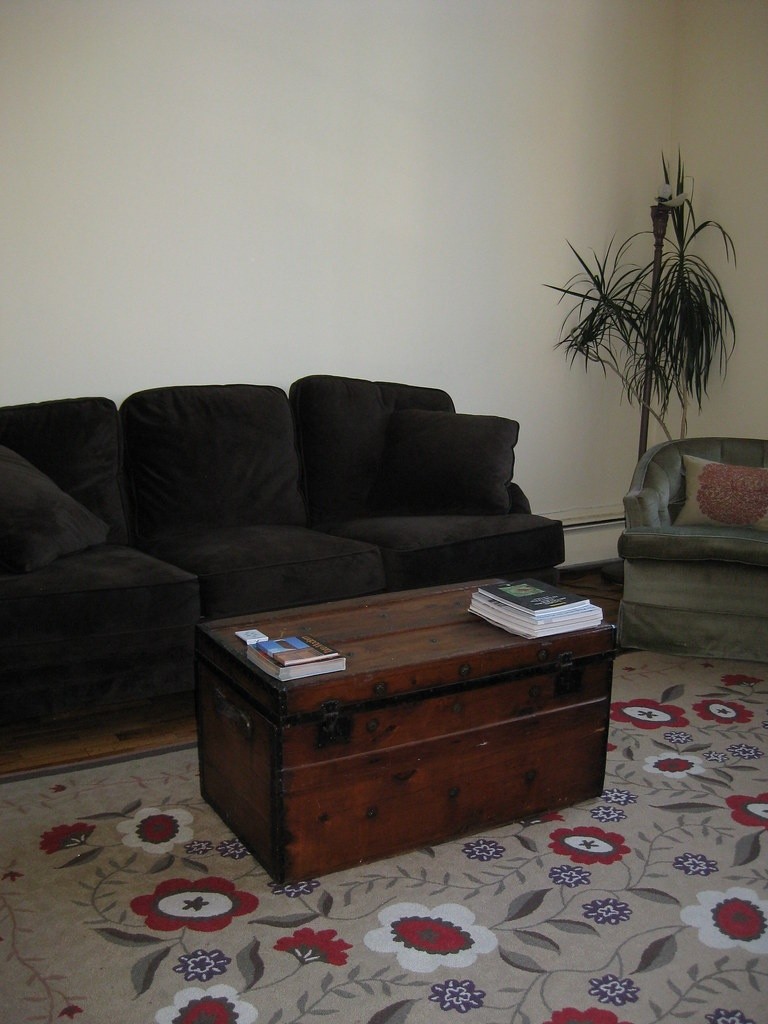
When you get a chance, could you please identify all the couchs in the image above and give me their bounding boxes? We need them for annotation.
[616,436,768,664]
[0,374,565,724]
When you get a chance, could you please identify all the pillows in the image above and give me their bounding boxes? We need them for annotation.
[364,410,520,516]
[0,444,111,574]
[669,455,768,533]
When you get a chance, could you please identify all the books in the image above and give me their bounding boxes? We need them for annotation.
[468,578,604,639]
[247,634,347,681]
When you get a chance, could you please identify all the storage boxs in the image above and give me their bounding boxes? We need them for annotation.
[196,577,618,883]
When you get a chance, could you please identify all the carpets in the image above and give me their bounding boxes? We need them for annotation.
[0,654,768,1024]
